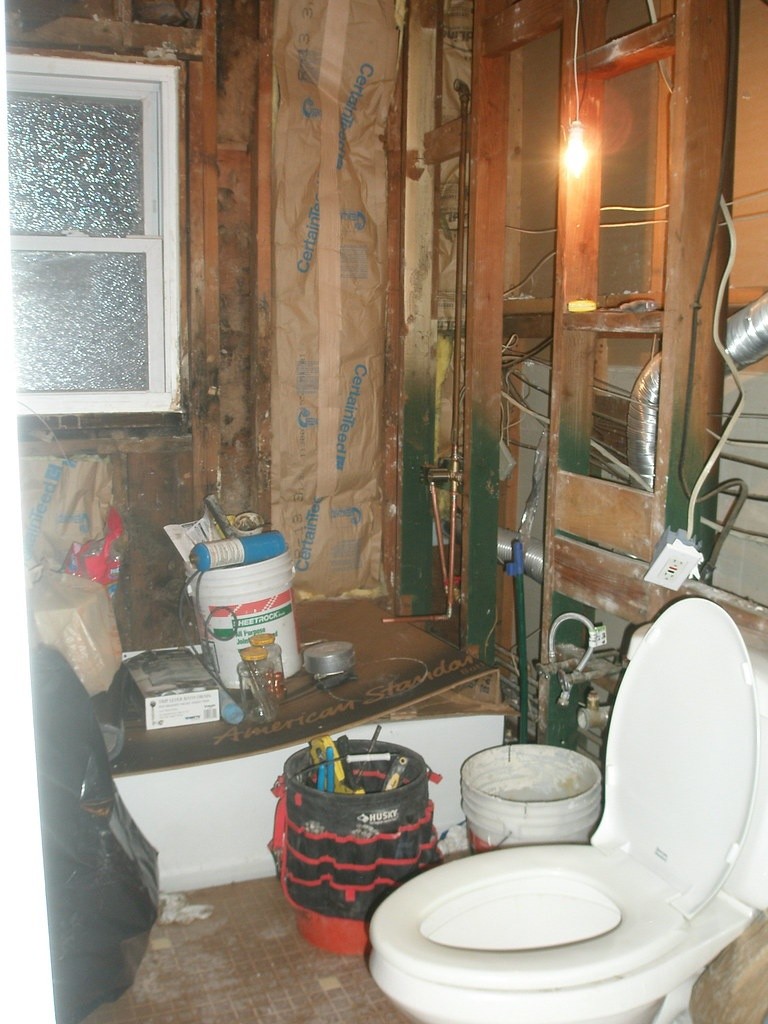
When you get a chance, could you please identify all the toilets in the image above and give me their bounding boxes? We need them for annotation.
[366,598,767,1021]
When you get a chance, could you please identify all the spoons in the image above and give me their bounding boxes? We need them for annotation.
[239,522,272,531]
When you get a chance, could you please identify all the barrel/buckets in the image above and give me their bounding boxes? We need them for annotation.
[282,726,444,956]
[460,744,602,855]
[185,550,302,690]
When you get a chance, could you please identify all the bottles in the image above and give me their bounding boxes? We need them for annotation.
[217,682,244,725]
[188,529,287,570]
[237,634,286,725]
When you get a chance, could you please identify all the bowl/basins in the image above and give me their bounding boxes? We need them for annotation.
[233,512,264,536]
